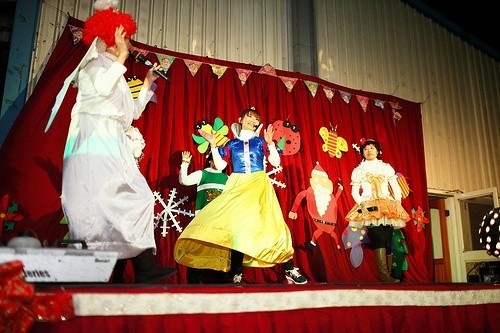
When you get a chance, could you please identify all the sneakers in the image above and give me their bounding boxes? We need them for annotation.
[284,265,307,284]
[231,269,242,286]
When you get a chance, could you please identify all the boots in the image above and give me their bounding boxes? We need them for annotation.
[375,248,400,283]
[386,255,392,275]
[112,259,127,283]
[131,249,176,283]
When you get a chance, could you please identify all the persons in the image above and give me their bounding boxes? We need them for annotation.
[350,139,411,283]
[173,109,307,287]
[45,8,178,284]
[178,149,230,284]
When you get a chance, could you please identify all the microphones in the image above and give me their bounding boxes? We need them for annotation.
[132,51,169,81]
